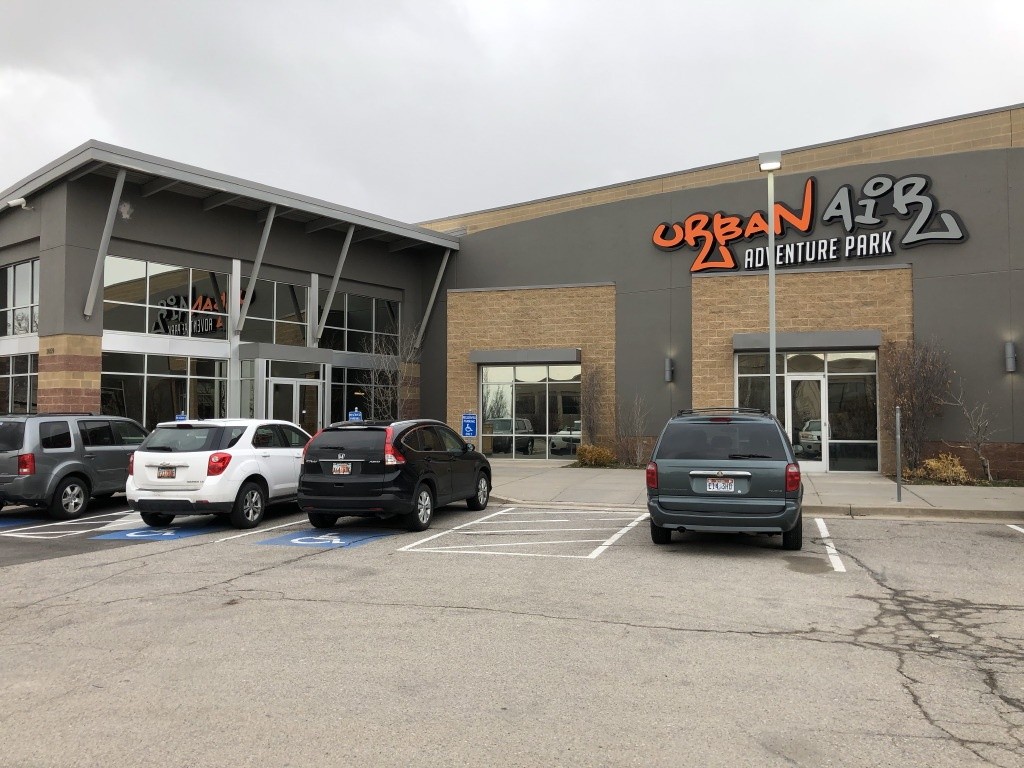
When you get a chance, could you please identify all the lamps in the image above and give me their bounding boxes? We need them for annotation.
[664,358,674,382]
[7,198,35,211]
[1005,342,1016,372]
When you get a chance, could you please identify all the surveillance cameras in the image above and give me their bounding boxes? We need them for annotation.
[8,198,26,208]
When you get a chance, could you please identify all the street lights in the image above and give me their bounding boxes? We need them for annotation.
[756,149,783,423]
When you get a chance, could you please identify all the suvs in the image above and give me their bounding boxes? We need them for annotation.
[295,419,492,532]
[645,407,804,552]
[485,418,536,455]
[126,419,312,530]
[0,412,152,519]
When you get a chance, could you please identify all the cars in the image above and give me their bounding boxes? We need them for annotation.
[798,419,833,453]
[550,421,583,456]
